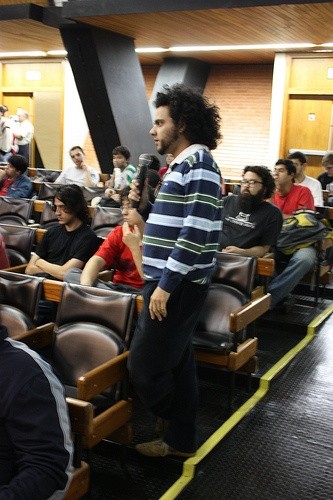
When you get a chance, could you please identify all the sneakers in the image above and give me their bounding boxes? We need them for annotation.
[135,438,196,457]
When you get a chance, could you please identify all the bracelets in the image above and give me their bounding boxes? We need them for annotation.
[33,258,41,266]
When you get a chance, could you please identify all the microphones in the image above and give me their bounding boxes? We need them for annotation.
[130,153,152,208]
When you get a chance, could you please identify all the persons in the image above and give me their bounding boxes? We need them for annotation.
[124,87,223,459]
[9,109,35,166]
[287,151,326,210]
[0,105,15,159]
[24,184,100,322]
[316,152,333,209]
[0,152,32,200]
[1,320,76,500]
[98,146,136,191]
[62,184,152,297]
[265,159,317,306]
[107,152,174,209]
[52,147,100,188]
[217,164,285,256]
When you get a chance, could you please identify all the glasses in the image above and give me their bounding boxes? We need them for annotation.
[272,168,288,174]
[239,179,263,187]
[120,203,136,211]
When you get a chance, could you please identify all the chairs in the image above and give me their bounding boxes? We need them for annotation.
[4,281,137,449]
[61,396,93,500]
[0,164,333,373]
